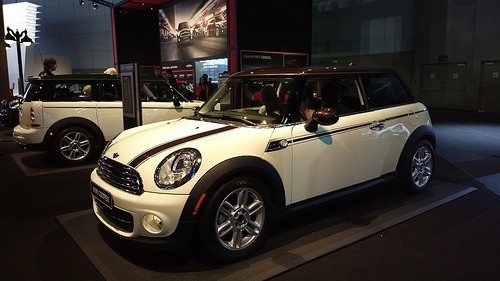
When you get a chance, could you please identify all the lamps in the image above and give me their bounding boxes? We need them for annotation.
[79,0,85,5]
[92,2,98,11]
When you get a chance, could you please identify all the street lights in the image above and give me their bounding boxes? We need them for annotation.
[2,27,34,97]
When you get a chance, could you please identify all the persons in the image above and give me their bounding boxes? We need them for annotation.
[152,68,164,80]
[259,85,317,122]
[38,57,57,76]
[166,70,178,90]
[196,74,211,101]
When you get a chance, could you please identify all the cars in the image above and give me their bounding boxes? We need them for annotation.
[0,93,24,128]
[89,66,438,263]
[175,15,227,48]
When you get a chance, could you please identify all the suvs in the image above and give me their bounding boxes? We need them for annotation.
[11,73,222,167]
